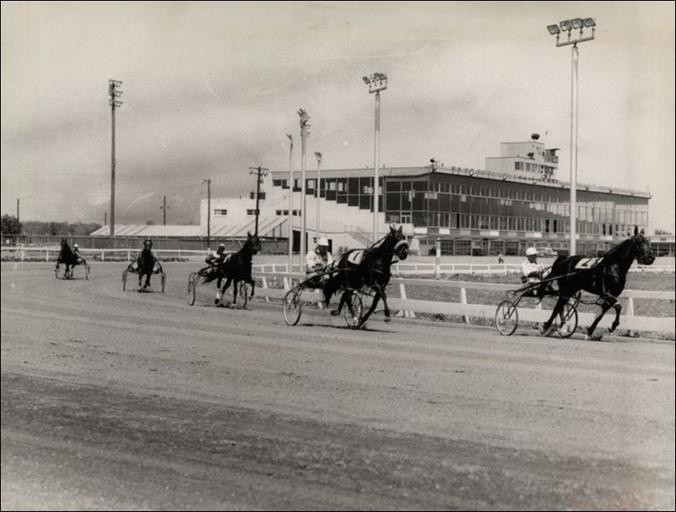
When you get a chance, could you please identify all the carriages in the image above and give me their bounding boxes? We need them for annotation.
[181,230,266,312]
[491,225,657,340]
[279,224,411,328]
[50,237,96,281]
[115,240,172,293]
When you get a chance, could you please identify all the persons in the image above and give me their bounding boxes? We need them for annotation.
[205,243,226,274]
[305,236,334,279]
[498,251,505,263]
[518,246,559,293]
[73,243,82,263]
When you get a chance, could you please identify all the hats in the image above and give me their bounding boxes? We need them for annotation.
[317,237,329,246]
[73,244,78,247]
[526,247,538,256]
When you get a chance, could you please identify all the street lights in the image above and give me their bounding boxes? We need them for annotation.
[284,132,294,275]
[544,16,604,260]
[296,106,311,280]
[105,77,122,237]
[314,149,325,246]
[362,71,389,248]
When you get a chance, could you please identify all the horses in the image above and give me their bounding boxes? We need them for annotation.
[137,238,154,291]
[323,223,410,328]
[202,230,259,307]
[54,237,78,279]
[535,225,655,340]
